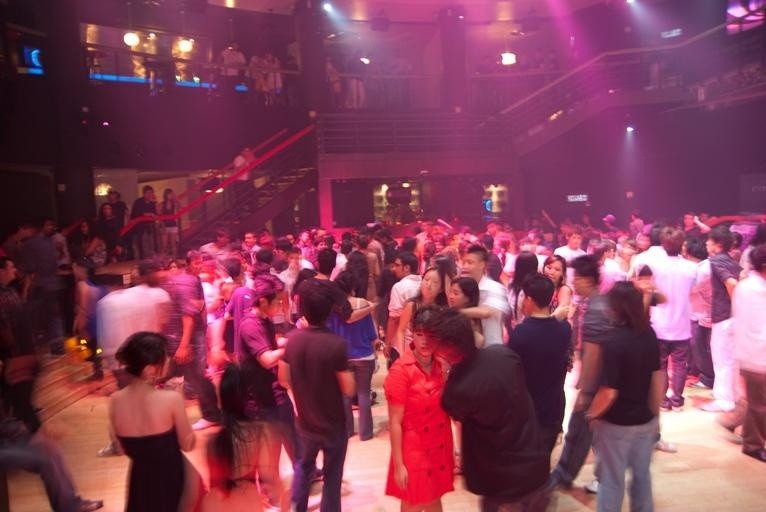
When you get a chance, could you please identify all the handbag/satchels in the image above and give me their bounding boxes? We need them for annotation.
[222,364,276,423]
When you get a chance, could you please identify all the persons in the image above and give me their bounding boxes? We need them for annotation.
[425,307,554,509]
[505,208,764,462]
[138,224,385,442]
[383,305,462,509]
[233,274,323,483]
[548,255,619,493]
[278,277,356,509]
[193,361,292,509]
[2,188,180,509]
[135,29,378,110]
[585,281,664,508]
[375,218,515,476]
[506,272,572,473]
[234,146,257,218]
[109,331,207,509]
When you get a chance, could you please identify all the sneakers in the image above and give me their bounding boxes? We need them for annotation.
[97,446,119,457]
[189,419,219,430]
[701,402,726,411]
[657,442,676,452]
[585,479,598,493]
[80,498,103,511]
[660,403,684,412]
